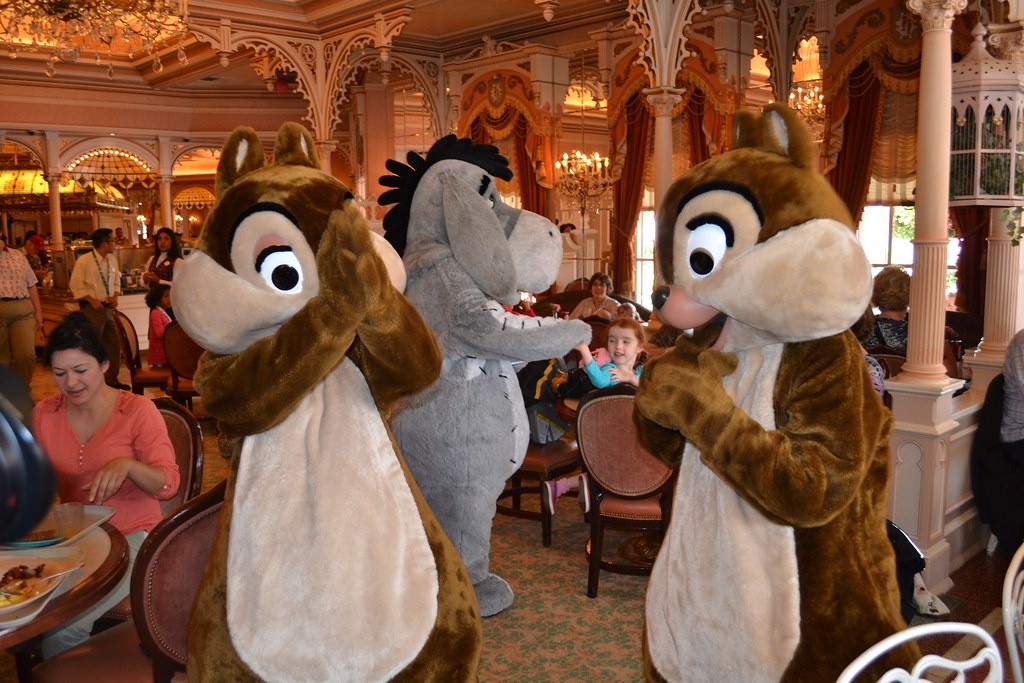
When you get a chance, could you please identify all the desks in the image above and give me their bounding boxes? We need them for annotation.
[0,523,130,678]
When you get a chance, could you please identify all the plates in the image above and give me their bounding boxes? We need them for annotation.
[0,505,116,628]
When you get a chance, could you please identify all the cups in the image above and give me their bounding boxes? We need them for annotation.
[53,502,84,539]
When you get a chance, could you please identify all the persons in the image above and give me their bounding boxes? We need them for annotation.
[137,227,189,389]
[113,227,130,246]
[964,330,1024,576]
[0,232,42,387]
[69,228,131,390]
[497,268,684,444]
[24,231,48,286]
[145,283,173,368]
[849,266,951,624]
[30,307,185,663]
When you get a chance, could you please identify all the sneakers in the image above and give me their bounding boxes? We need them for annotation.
[578,472,591,513]
[542,480,558,516]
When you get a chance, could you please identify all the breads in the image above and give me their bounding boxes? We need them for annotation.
[18,530,57,542]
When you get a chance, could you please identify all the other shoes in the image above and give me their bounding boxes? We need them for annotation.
[113,383,130,390]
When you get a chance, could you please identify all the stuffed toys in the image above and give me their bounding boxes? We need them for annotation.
[170,123,484,683]
[373,132,593,619]
[627,104,924,683]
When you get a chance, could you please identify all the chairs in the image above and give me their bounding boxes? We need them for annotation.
[100,398,206,622]
[109,309,171,394]
[161,320,219,431]
[574,382,685,597]
[495,440,592,546]
[30,478,228,683]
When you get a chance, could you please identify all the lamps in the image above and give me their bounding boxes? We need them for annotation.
[0,0,190,79]
[556,151,608,220]
[789,86,826,125]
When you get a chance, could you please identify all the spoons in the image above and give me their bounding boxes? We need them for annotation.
[0,563,86,595]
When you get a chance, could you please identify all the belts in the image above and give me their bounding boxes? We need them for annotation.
[1,297,19,301]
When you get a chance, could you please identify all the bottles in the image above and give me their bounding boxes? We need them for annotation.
[560,312,571,321]
